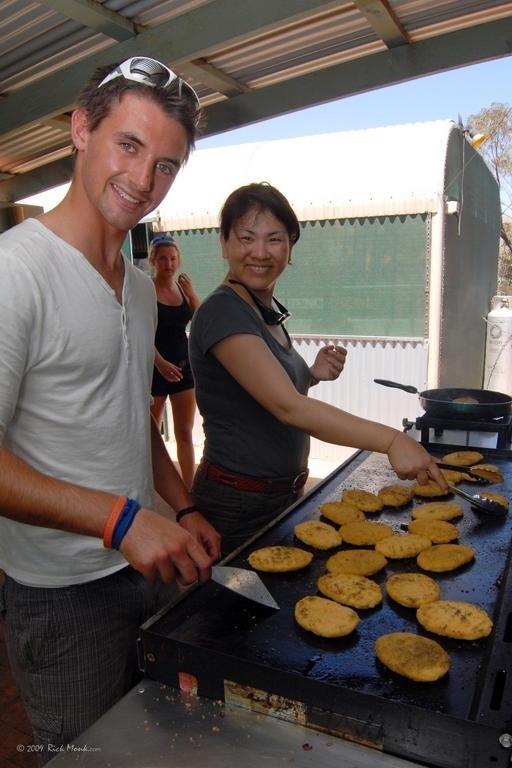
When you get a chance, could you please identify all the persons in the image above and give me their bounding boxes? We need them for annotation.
[189,182,449,557]
[0,54,222,768]
[149,235,202,489]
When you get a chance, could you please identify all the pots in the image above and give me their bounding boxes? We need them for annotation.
[372,378,512,419]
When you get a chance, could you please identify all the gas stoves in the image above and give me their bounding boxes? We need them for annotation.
[415,412,512,449]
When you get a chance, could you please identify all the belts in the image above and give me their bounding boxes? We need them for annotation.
[197,456,309,495]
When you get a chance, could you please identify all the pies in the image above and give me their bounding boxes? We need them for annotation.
[453,397,478,405]
[247,450,509,682]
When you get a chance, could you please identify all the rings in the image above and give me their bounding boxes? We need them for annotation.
[170,372,174,374]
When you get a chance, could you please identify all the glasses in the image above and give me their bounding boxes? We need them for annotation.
[96,56,203,127]
[228,279,291,327]
[151,236,176,245]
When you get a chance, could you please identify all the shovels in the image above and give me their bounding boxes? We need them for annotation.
[195,566,280,609]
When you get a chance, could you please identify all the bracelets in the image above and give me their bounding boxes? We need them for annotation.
[386,431,400,453]
[176,506,198,521]
[112,499,142,551]
[103,495,127,548]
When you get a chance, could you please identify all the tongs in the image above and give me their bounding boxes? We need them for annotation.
[426,462,508,519]
